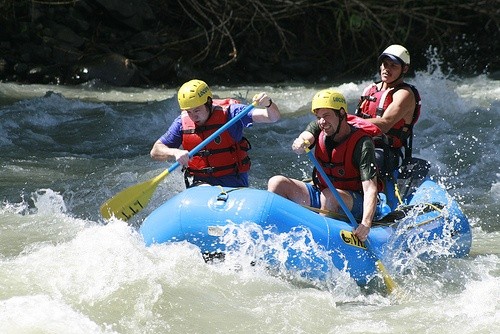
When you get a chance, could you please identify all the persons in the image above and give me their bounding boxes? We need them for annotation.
[150,78,280,187]
[355,44,421,201]
[267,90,377,241]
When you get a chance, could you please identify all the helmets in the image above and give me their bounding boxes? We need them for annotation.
[310,89,348,115]
[382,45,412,62]
[178,79,213,109]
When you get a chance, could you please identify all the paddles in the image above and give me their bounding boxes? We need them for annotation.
[302,146,402,301]
[98,100,262,224]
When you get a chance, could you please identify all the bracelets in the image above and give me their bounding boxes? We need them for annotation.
[266,99,272,107]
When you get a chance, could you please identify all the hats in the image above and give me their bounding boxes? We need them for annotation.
[377,54,401,64]
